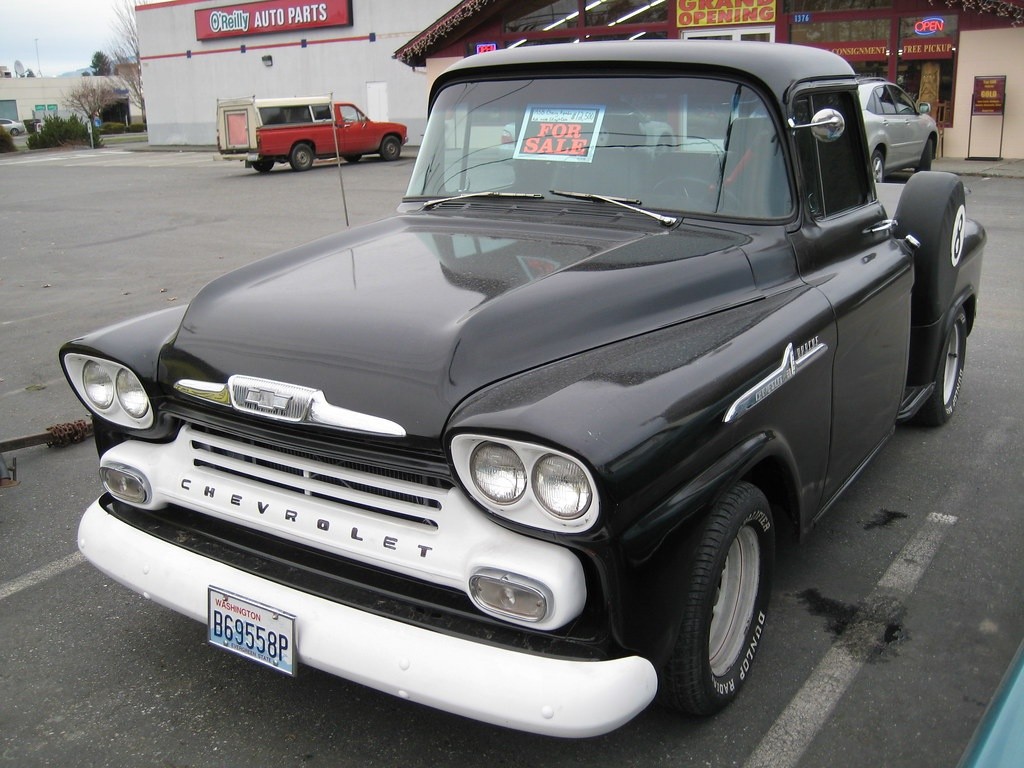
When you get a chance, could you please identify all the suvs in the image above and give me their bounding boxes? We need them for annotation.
[60,37,987,741]
[852,77,941,183]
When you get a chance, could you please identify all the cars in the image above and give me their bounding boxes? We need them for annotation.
[0,118,25,136]
[497,96,675,180]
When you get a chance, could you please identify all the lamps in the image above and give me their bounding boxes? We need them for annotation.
[262,56,272,66]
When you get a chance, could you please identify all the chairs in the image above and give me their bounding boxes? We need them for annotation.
[707,117,791,218]
[552,115,650,197]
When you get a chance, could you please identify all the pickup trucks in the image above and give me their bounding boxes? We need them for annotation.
[215,91,408,171]
